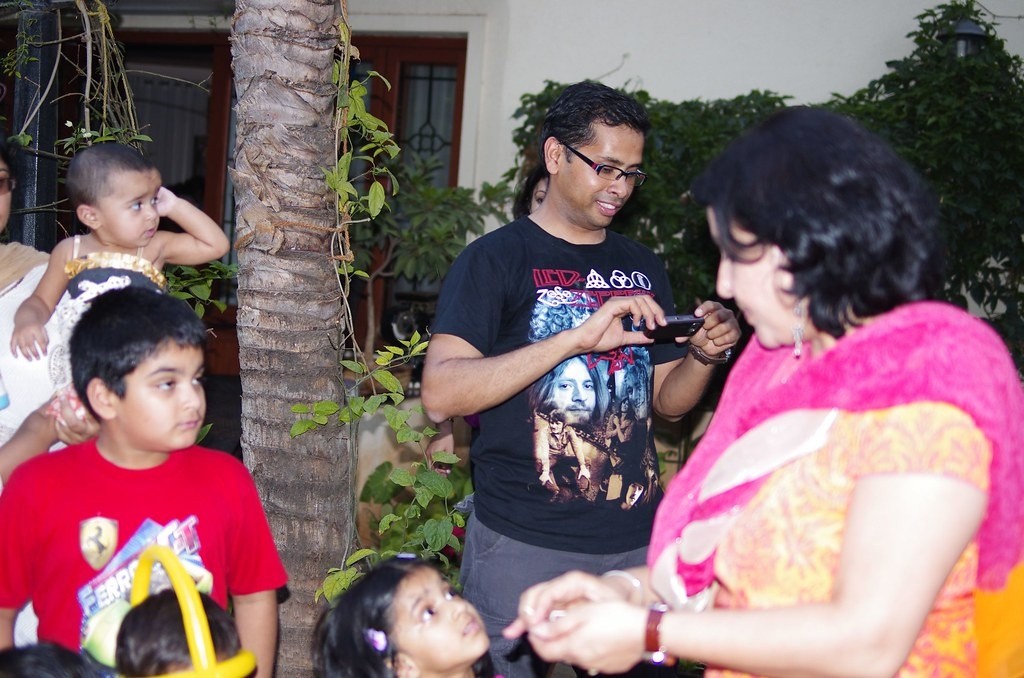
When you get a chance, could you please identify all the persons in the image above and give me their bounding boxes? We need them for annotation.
[0,80,1024,678]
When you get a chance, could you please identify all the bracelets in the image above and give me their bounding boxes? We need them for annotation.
[602,570,647,609]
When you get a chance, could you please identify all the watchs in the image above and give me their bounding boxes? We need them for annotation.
[642,604,676,668]
[688,342,732,366]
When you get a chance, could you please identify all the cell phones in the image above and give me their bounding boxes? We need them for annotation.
[643,314,705,339]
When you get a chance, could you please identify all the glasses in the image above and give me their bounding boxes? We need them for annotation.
[557,138,648,187]
